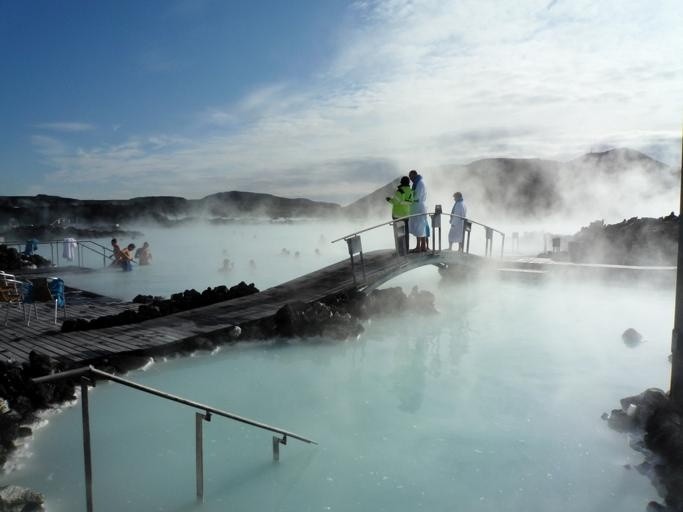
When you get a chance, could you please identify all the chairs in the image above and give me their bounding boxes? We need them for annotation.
[17,276,65,325]
[6,279,34,327]
[0,270,23,325]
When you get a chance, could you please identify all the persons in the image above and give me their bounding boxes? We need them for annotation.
[108,233,326,277]
[384,175,413,257]
[408,171,429,252]
[445,191,468,251]
